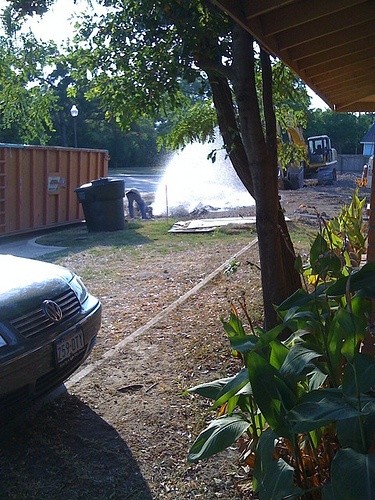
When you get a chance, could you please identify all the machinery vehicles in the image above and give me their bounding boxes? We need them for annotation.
[289,135,339,189]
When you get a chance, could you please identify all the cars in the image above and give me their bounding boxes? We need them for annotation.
[0,253,103,412]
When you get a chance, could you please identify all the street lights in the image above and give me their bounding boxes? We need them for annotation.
[70,104,78,148]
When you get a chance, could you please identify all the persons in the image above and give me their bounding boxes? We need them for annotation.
[315,145,323,155]
[126,187,153,219]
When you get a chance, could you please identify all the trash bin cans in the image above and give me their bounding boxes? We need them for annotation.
[76,177,126,233]
